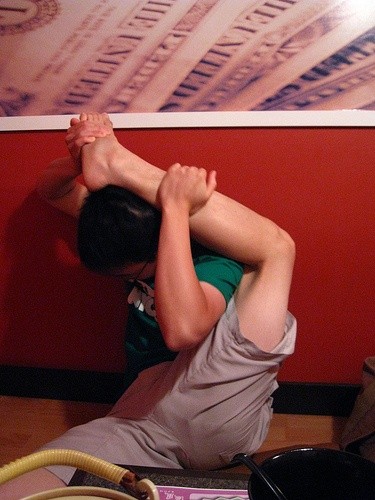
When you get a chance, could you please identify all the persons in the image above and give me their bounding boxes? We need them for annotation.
[0,111,298,499]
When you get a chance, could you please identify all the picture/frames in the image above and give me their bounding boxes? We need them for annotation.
[1,0,375,133]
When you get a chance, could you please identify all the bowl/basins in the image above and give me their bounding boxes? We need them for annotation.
[249,446,374,500]
